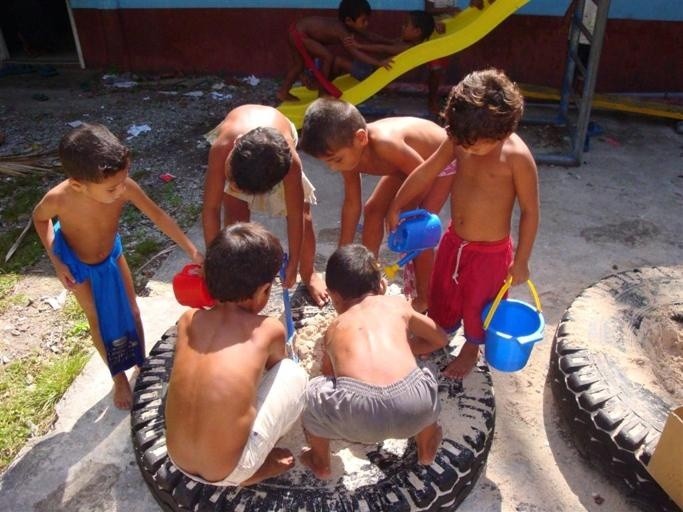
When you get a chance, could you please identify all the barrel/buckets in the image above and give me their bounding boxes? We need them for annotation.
[481,276,545,372]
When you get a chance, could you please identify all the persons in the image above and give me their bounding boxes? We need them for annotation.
[276,0,395,100]
[564,0,607,98]
[329,10,436,84]
[468,0,506,9]
[386,69,539,378]
[298,244,448,478]
[299,57,323,85]
[298,96,458,312]
[32,123,205,409]
[165,223,310,487]
[423,0,461,117]
[202,103,332,306]
